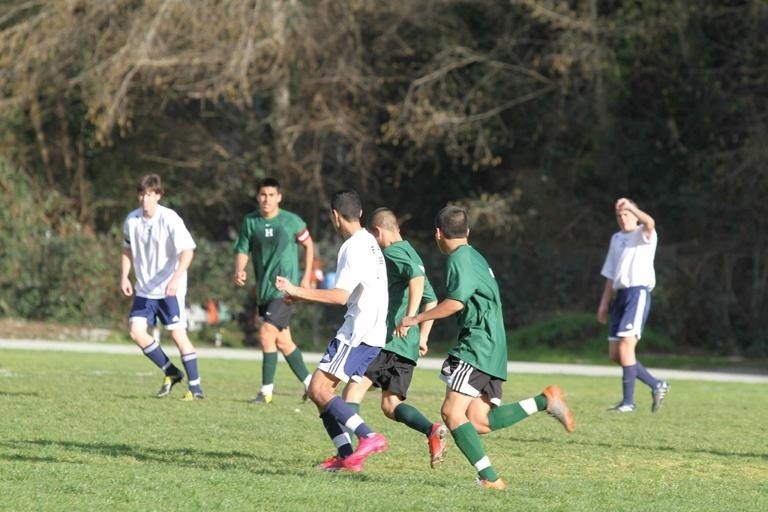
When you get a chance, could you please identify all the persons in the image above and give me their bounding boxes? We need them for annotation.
[342,206,448,470]
[597,197,671,413]
[274,189,389,471]
[232,178,314,404]
[401,205,574,488]
[119,172,204,400]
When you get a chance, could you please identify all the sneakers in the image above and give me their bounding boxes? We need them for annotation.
[427,421,449,470]
[608,402,636,414]
[651,379,670,413]
[157,369,185,400]
[342,432,388,468]
[248,391,274,405]
[470,477,506,492]
[542,383,574,433]
[315,456,362,472]
[178,390,204,402]
[300,390,312,405]
